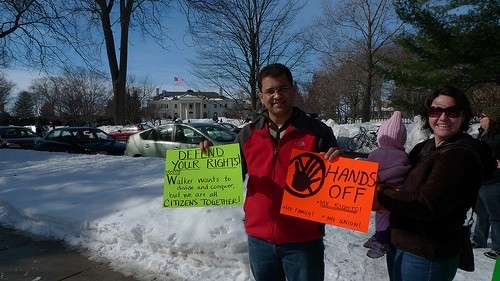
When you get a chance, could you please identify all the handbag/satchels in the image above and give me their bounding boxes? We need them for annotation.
[457,219,474,272]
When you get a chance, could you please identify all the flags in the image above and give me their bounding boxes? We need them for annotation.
[174,76,184,86]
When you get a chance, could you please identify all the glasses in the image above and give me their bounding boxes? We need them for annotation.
[262,85,293,96]
[480,114,487,118]
[425,106,464,119]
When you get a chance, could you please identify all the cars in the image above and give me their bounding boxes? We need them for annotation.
[0,120,241,157]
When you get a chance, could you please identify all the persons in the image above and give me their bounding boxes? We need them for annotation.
[353,109,409,260]
[239,115,252,124]
[133,112,191,143]
[213,111,219,123]
[464,107,500,260]
[385,87,497,281]
[33,120,56,138]
[198,63,343,281]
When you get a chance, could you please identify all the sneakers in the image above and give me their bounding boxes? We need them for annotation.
[471,239,484,248]
[367,242,388,259]
[483,249,500,259]
[363,233,380,248]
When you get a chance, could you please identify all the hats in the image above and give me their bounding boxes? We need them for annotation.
[376,111,408,151]
[482,106,500,120]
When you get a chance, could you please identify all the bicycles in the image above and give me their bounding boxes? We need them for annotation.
[347,125,379,150]
